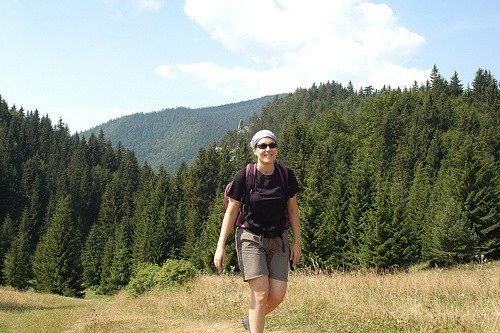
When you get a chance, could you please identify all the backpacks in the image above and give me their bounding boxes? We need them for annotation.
[224,162,289,228]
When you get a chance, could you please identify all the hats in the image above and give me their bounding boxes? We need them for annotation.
[249,129,278,148]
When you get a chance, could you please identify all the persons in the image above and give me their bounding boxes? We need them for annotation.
[212,129,302,333]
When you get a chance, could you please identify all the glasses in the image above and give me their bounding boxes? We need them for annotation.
[256,143,277,149]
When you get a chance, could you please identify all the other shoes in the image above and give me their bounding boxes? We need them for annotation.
[243,316,249,331]
